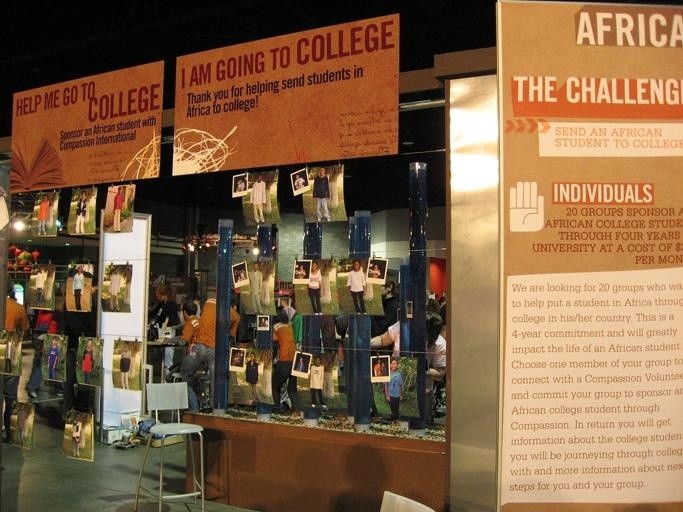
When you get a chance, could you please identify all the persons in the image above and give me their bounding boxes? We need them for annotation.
[145,282,240,412]
[5,186,132,458]
[229,275,448,421]
[234,262,263,314]
[293,168,331,221]
[295,261,324,315]
[234,173,266,224]
[346,261,381,314]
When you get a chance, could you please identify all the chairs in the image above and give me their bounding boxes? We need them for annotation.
[379,490,436,512]
[133,382,205,512]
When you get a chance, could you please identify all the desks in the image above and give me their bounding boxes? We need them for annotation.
[147,340,189,383]
